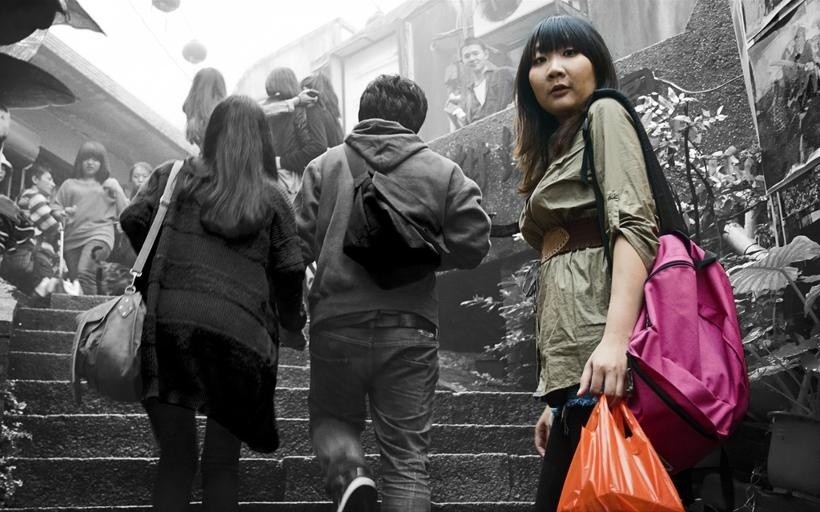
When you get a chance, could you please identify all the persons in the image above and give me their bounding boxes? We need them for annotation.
[0,108,154,331]
[443,44,531,129]
[182,68,319,158]
[259,67,331,185]
[292,74,492,511]
[508,13,699,510]
[120,95,309,512]
[265,72,347,173]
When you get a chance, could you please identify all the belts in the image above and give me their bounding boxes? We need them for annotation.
[347,311,437,330]
[538,221,602,264]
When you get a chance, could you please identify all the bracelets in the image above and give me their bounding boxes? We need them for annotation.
[297,96,304,107]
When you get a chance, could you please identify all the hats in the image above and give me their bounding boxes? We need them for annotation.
[0,153,13,172]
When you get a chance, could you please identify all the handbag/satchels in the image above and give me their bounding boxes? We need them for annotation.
[1,250,35,276]
[72,293,144,403]
[343,170,448,290]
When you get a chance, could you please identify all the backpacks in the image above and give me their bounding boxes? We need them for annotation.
[582,88,750,477]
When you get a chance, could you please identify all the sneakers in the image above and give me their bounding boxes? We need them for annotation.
[335,468,378,512]
